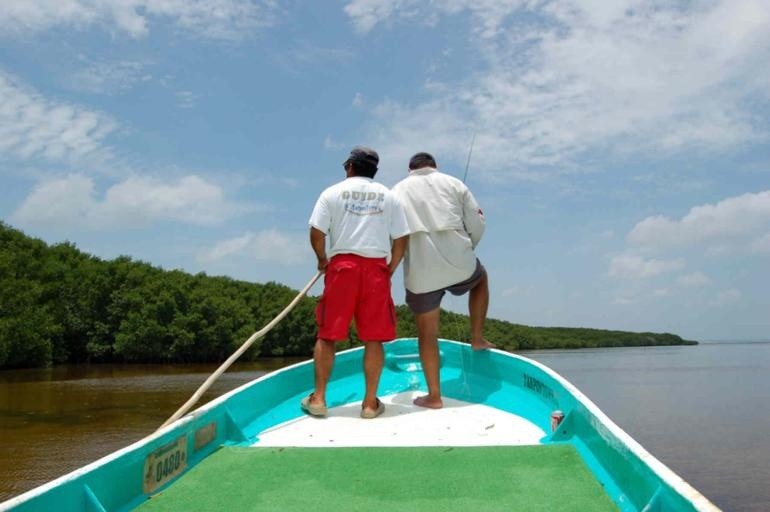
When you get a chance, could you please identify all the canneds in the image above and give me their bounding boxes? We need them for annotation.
[551,410,564,432]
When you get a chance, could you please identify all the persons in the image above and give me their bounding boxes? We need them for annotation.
[301,146,411,419]
[387,153,497,408]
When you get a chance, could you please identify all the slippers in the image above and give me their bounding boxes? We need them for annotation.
[300,393,327,417]
[360,397,385,419]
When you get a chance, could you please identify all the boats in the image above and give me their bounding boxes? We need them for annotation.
[0,334,723,512]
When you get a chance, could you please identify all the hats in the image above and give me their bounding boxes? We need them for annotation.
[342,146,379,171]
